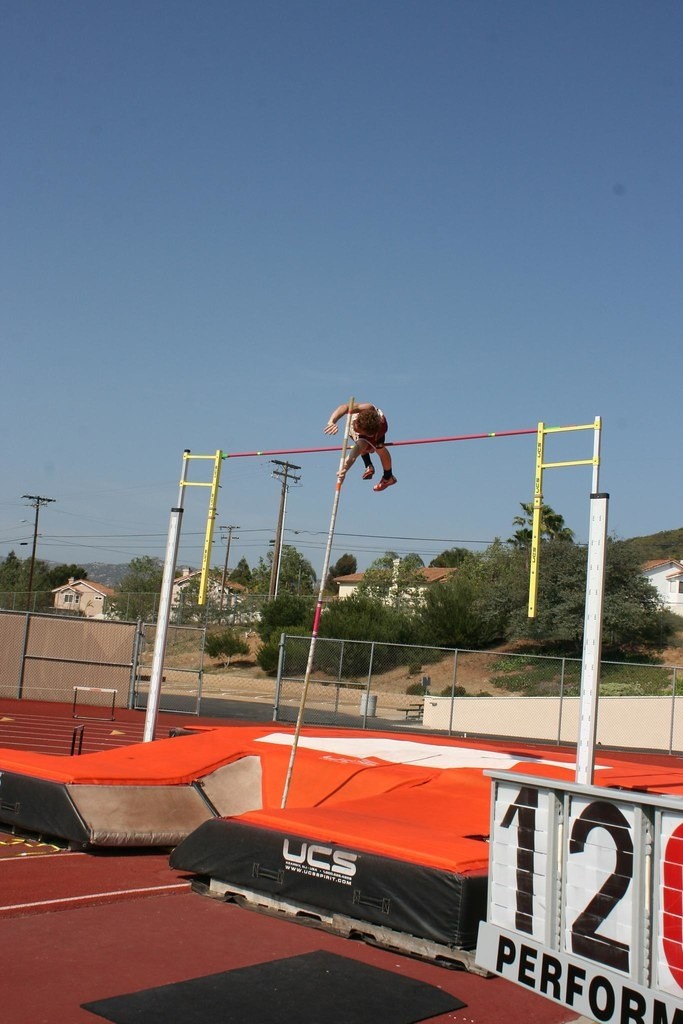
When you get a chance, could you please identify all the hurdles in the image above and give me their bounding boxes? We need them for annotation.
[69,725,86,756]
[71,685,118,720]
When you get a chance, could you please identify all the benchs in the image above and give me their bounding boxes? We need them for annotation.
[396,708,424,719]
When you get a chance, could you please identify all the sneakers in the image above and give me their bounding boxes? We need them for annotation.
[373,475,397,491]
[362,466,375,480]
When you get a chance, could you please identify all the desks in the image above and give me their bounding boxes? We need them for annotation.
[409,704,424,716]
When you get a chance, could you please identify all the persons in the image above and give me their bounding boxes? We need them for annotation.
[325,402,398,492]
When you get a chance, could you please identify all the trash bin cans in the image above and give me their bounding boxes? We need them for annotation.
[359,693,378,717]
